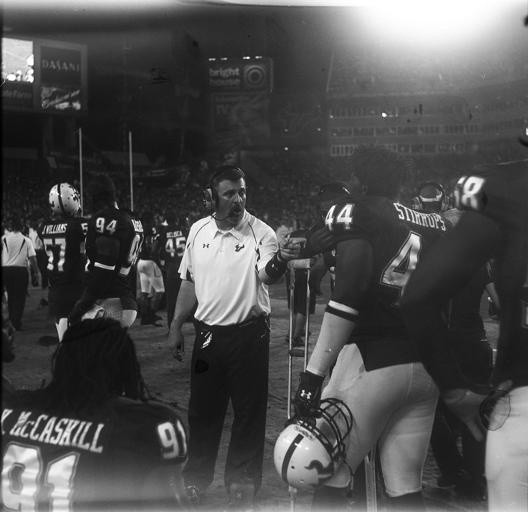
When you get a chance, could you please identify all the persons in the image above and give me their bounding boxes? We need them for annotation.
[150,210,190,337]
[292,143,445,508]
[67,174,144,331]
[445,123,528,508]
[0,301,17,403]
[135,212,166,326]
[0,317,188,507]
[422,259,496,501]
[32,182,89,343]
[1,216,41,333]
[284,211,325,348]
[167,164,303,507]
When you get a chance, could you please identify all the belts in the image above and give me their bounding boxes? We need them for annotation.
[193,321,258,335]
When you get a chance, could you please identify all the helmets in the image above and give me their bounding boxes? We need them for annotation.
[273,396,354,492]
[49,181,81,218]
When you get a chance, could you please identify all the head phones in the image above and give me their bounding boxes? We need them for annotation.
[201,166,237,223]
